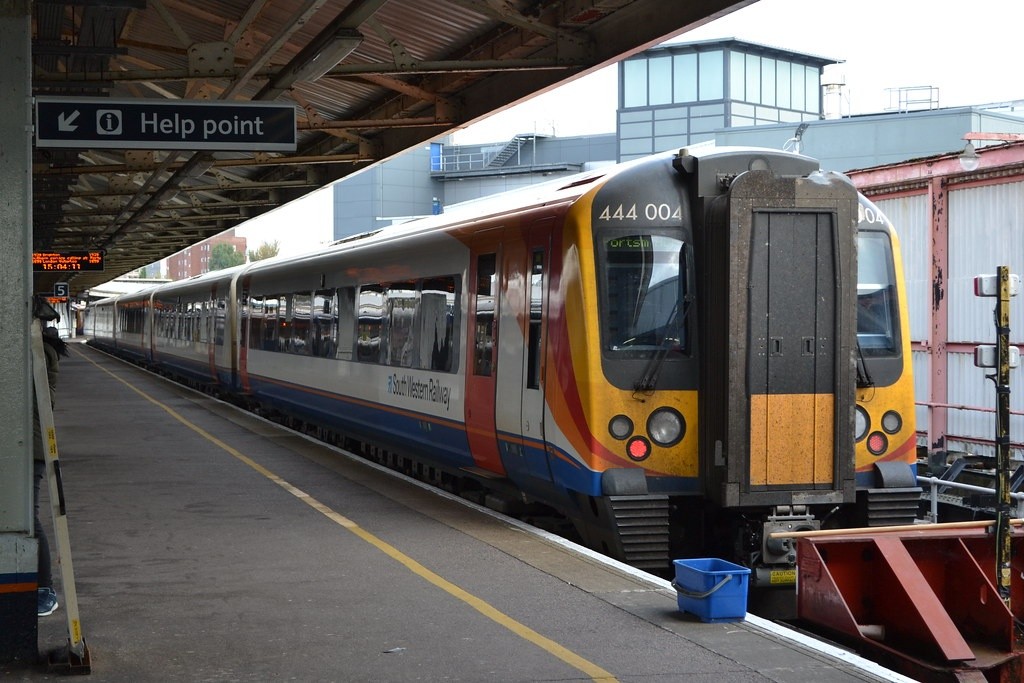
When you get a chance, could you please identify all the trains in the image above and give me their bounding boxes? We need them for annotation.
[83,148,924,622]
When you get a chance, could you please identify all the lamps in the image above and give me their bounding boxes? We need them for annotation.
[292,27,364,83]
[960,133,1024,171]
[106,209,156,249]
[156,183,181,200]
[186,155,216,179]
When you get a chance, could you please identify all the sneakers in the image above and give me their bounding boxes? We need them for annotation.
[37,588,58,616]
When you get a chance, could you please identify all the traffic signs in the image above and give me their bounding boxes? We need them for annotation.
[34,94,297,150]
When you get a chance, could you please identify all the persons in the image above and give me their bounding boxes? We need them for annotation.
[33,295,69,617]
